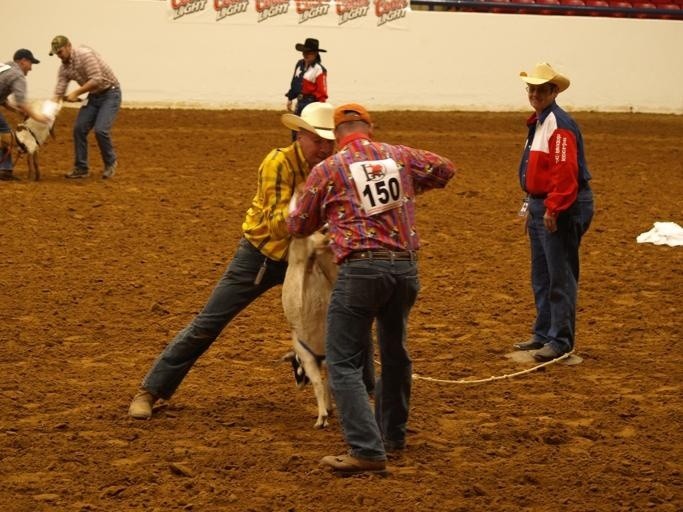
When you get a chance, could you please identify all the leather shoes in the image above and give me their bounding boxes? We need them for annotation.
[0,170,20,180]
[129,390,154,417]
[513,339,543,349]
[323,454,386,470]
[534,344,558,360]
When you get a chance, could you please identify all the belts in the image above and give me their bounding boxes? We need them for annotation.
[348,253,416,259]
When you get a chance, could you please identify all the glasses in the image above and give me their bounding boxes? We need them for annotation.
[526,86,549,94]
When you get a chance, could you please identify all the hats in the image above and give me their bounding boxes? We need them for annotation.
[296,38,326,52]
[334,104,371,126]
[49,36,68,55]
[281,102,336,140]
[13,48,40,63]
[520,62,570,92]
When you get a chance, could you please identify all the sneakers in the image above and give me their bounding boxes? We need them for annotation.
[103,160,117,178]
[65,169,89,178]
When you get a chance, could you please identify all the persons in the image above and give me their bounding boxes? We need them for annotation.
[284,103,456,475]
[283,36,328,142]
[44,32,123,180]
[127,101,379,421]
[512,60,594,362]
[0,46,50,184]
